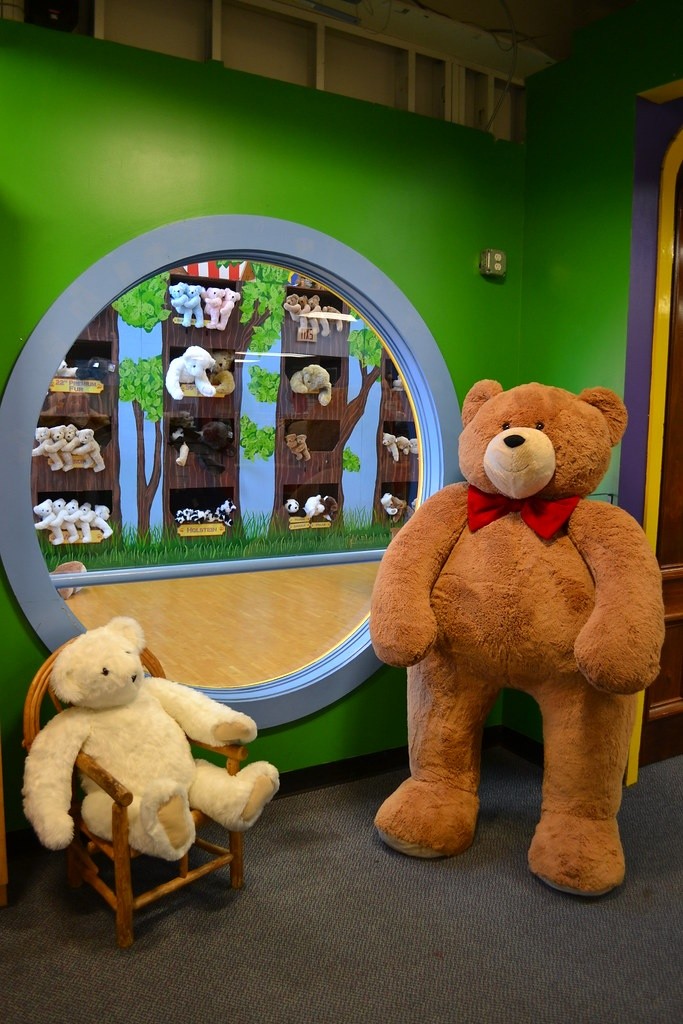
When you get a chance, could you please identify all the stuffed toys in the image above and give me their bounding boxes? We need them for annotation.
[290,365,332,406]
[168,282,192,327]
[31,412,407,545]
[308,295,330,337]
[283,294,301,322]
[184,283,203,328]
[56,357,111,380]
[382,433,399,461]
[208,350,235,394]
[298,295,319,334]
[322,306,343,331]
[395,436,410,456]
[201,287,224,329]
[372,378,666,897]
[21,618,280,861]
[409,439,419,454]
[49,561,87,599]
[167,346,216,399]
[218,288,241,330]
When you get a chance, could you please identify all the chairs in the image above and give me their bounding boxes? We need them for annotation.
[22,632,248,948]
